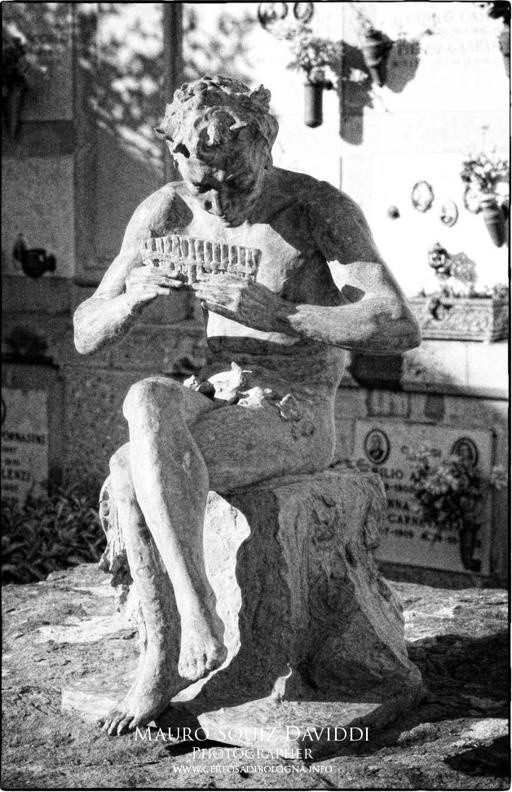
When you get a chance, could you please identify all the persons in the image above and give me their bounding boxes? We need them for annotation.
[72,76,423,735]
